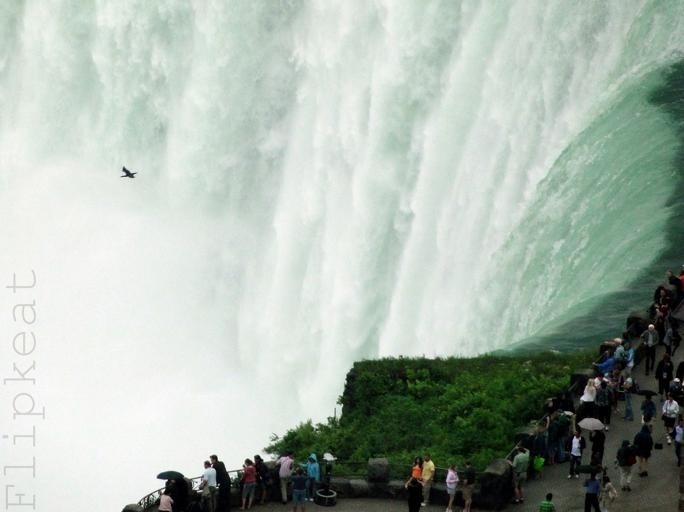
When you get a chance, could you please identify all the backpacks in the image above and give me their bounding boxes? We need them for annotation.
[643,401,653,422]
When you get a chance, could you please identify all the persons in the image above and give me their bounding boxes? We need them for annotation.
[505,266,683,511]
[154,448,476,511]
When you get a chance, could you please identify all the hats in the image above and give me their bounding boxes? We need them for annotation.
[673,378,680,382]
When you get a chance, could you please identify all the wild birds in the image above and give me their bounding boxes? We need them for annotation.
[121,166,137,179]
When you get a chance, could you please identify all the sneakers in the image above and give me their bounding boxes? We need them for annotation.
[567,473,580,479]
[511,496,525,504]
[420,501,431,507]
[638,471,649,478]
[622,487,632,492]
[445,508,471,512]
